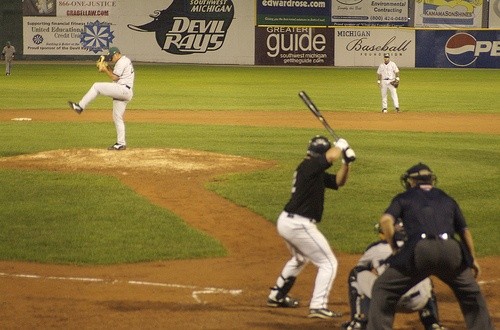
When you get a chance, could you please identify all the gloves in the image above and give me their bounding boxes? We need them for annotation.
[333,138,348,151]
[341,149,356,165]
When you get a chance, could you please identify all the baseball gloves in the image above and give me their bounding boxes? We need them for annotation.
[390,79,399,88]
[96,55,109,73]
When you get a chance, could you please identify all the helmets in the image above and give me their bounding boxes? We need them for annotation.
[306,136,331,158]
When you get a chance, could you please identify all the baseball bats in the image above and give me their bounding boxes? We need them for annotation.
[297,91,356,162]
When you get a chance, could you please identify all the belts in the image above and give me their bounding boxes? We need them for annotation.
[126,85,131,89]
[416,233,449,240]
[287,213,293,217]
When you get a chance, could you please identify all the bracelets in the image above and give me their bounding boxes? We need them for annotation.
[396,77,400,81]
[377,80,380,83]
[378,79,380,80]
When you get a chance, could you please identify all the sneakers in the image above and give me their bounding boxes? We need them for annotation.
[68,101,83,114]
[308,309,342,319]
[108,143,126,150]
[266,295,298,307]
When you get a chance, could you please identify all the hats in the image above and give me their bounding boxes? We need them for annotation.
[385,54,389,57]
[107,47,120,59]
[403,162,431,177]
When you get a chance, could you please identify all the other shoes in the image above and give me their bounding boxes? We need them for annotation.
[396,108,400,112]
[382,108,386,112]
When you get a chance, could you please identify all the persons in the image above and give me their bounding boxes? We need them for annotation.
[68,47,134,150]
[342,217,450,330]
[366,162,493,330]
[267,135,357,319]
[377,53,401,113]
[2,41,15,75]
